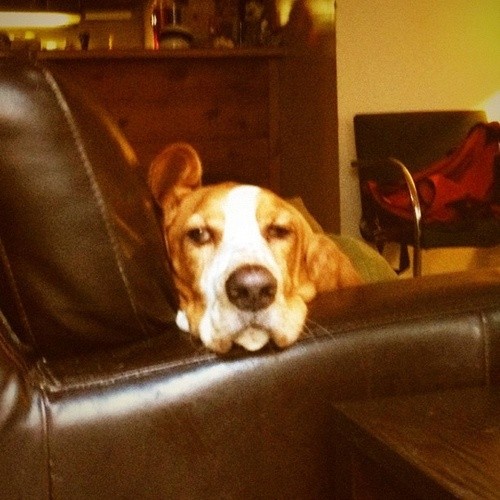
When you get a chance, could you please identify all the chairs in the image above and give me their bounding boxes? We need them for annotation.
[348,110,500,282]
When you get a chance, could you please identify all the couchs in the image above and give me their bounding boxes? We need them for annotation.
[0,55,500,500]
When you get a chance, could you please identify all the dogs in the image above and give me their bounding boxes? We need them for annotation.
[149,139,401,354]
